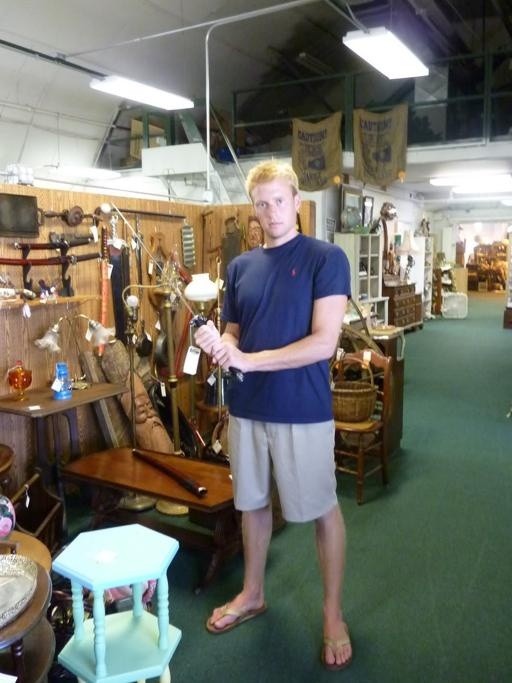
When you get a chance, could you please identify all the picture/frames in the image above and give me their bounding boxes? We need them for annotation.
[362,194,373,228]
[338,182,363,232]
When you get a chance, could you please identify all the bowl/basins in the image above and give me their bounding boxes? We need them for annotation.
[0,287,17,298]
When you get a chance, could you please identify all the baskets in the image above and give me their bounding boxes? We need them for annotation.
[330,357,378,423]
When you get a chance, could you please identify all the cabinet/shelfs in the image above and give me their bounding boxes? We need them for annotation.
[340,322,403,461]
[0,526,56,682]
[0,379,132,537]
[51,524,186,683]
[330,226,435,337]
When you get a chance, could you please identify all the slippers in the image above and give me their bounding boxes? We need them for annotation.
[206,599,266,634]
[321,621,353,671]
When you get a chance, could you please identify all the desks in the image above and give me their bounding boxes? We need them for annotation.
[62,443,245,596]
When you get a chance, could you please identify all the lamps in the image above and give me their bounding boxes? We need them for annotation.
[28,307,116,391]
[87,69,197,115]
[179,272,222,371]
[341,1,429,84]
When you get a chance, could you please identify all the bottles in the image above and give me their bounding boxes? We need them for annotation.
[53,361,73,400]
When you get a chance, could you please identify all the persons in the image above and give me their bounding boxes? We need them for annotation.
[195,158,353,671]
[119,370,174,453]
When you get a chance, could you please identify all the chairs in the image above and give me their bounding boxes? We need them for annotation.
[334,345,394,506]
